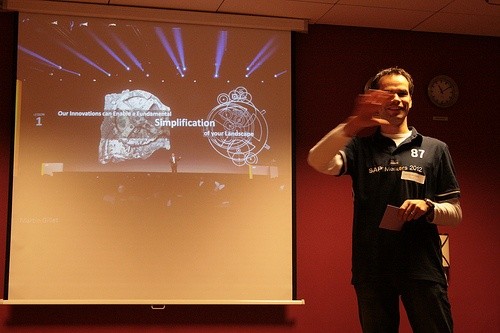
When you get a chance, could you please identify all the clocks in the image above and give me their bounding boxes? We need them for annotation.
[427,74,459,108]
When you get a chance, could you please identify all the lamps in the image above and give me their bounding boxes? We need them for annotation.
[438,234,451,289]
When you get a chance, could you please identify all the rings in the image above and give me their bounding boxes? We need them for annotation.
[411,212,415,216]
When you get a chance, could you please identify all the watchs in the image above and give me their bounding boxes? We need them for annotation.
[424,198,435,218]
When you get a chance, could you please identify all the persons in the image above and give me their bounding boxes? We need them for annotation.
[307,67,462,333]
